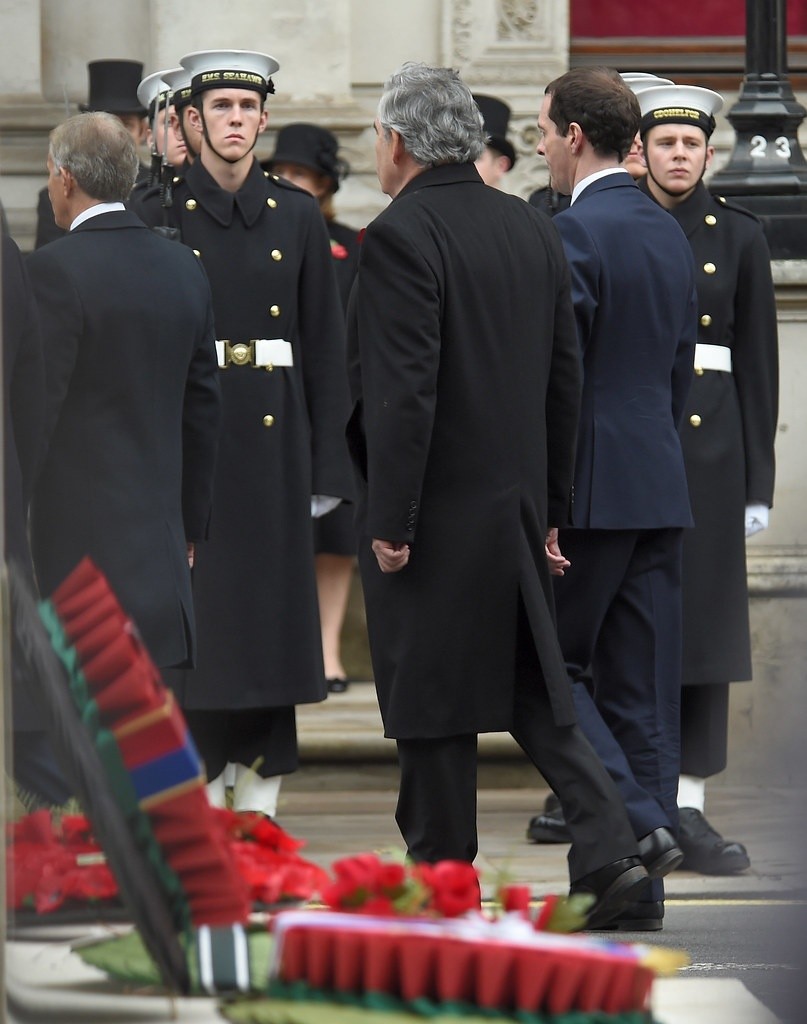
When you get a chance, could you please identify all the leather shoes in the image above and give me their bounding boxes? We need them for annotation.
[527,814,571,843]
[677,805,750,870]
[611,901,664,932]
[566,856,647,930]
[638,829,685,875]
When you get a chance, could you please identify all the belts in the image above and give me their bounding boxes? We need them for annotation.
[215,339,295,369]
[695,342,733,374]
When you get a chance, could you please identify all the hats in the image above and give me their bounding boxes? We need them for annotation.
[259,124,339,176]
[635,84,725,137]
[137,71,174,122]
[79,59,149,118]
[471,95,515,173]
[623,77,674,95]
[161,68,195,112]
[179,50,279,106]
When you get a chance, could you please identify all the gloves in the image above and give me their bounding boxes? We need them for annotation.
[312,496,343,519]
[745,502,769,539]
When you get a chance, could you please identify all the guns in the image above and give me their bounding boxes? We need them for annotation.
[153,90,180,241]
[146,85,163,188]
[546,176,561,218]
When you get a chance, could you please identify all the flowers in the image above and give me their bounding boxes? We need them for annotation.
[11,552,662,1024]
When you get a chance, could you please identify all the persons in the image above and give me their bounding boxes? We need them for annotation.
[2,49,782,928]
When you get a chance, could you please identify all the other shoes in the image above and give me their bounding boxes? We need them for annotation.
[329,679,348,693]
